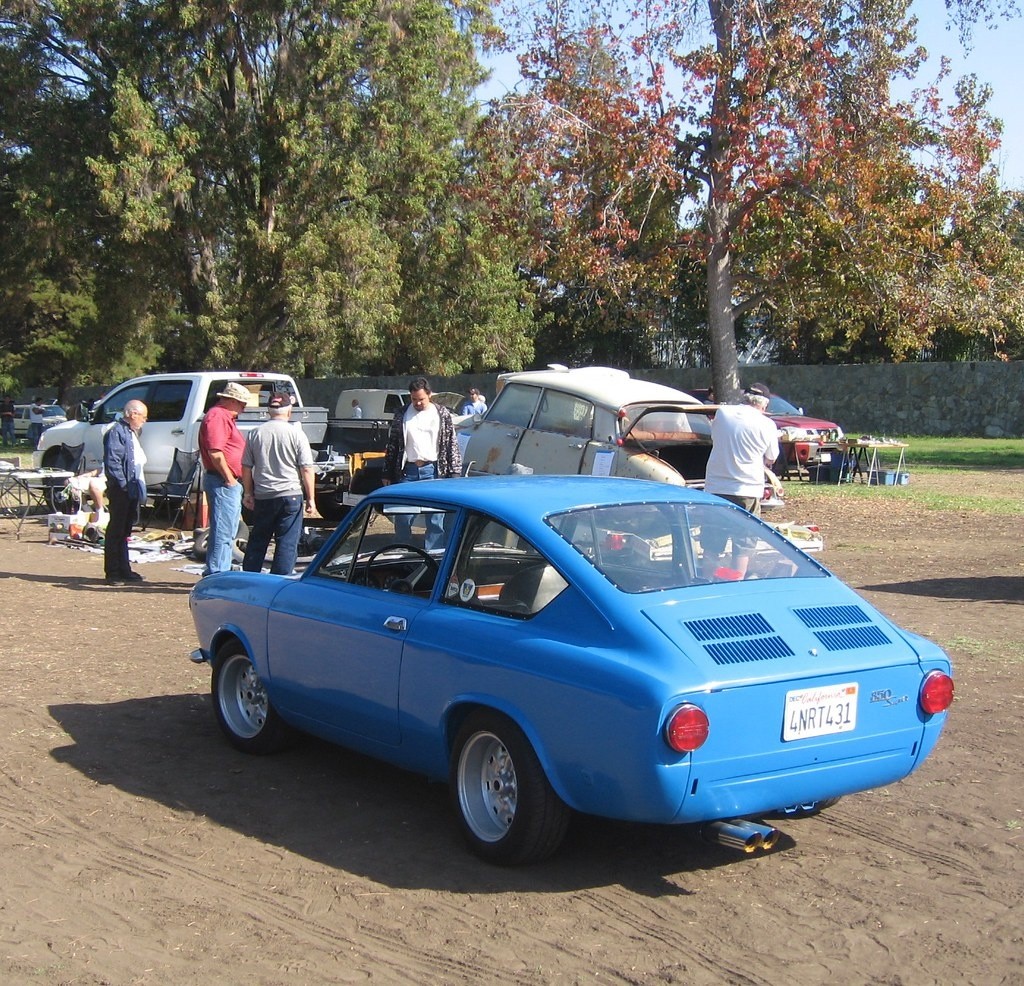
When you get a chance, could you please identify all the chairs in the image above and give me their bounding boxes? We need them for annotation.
[31,442,85,515]
[0,457,24,505]
[73,481,109,514]
[140,447,200,532]
[499,563,569,615]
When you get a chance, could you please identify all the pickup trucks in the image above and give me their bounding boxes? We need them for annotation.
[35,372,390,524]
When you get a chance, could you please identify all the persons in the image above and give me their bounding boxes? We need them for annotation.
[0,395,17,443]
[64,399,148,586]
[352,399,362,418]
[459,388,487,415]
[30,397,43,450]
[382,377,462,550]
[198,382,250,577]
[241,393,316,575]
[699,383,780,580]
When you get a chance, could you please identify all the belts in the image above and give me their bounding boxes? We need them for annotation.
[405,460,438,467]
[207,469,220,476]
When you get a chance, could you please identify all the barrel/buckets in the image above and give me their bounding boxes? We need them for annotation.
[829,452,855,483]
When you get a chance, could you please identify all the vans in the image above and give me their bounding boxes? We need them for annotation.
[336,389,411,419]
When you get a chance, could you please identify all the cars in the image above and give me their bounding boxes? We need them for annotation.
[189,476,954,870]
[689,387,846,476]
[460,374,782,522]
[13,405,67,437]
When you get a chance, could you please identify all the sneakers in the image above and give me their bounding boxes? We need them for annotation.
[105,571,144,586]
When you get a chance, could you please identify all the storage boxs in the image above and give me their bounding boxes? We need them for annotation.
[242,384,262,408]
[183,492,209,531]
[808,466,826,481]
[77,511,110,539]
[894,474,909,485]
[865,470,894,485]
[781,434,792,441]
[48,514,84,540]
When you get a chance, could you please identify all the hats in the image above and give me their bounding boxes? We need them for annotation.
[468,387,480,396]
[744,382,771,400]
[267,392,291,409]
[216,382,251,404]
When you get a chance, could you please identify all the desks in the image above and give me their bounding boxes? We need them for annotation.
[779,439,803,481]
[837,444,909,487]
[0,469,75,540]
